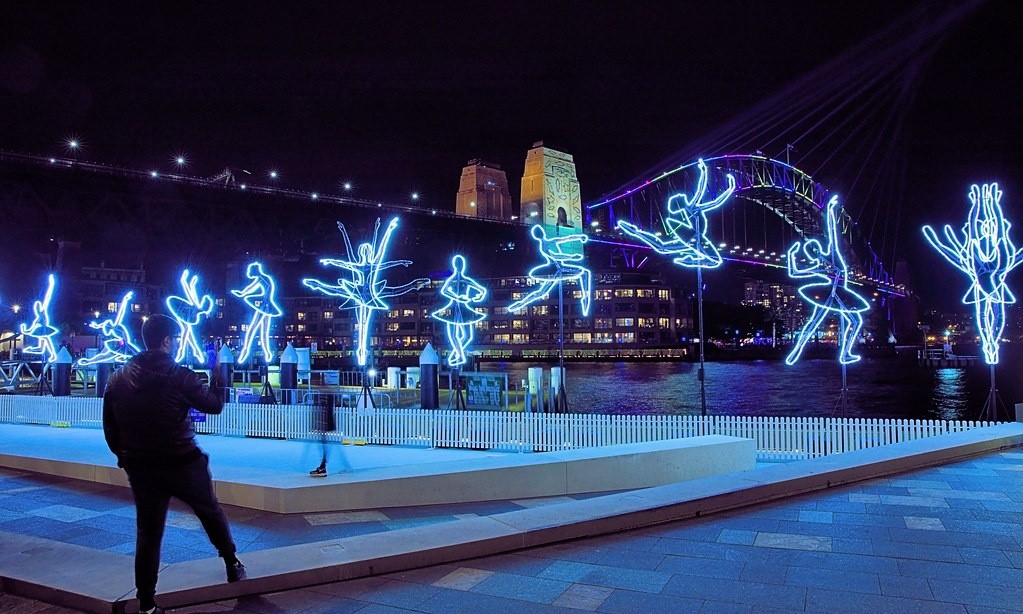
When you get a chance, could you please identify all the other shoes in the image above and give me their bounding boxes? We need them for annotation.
[223,554,245,583]
[139,606,165,614]
[309,468,327,478]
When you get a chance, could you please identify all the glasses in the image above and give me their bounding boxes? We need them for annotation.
[172,336,182,343]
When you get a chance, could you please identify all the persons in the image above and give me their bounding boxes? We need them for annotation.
[304,340,336,477]
[102,314,247,614]
